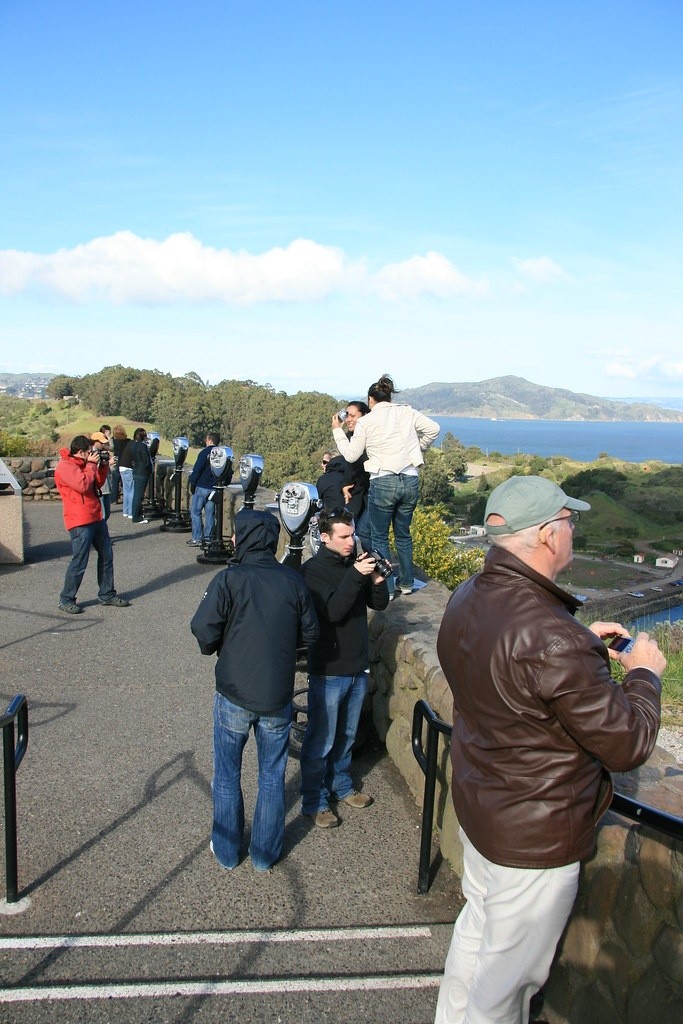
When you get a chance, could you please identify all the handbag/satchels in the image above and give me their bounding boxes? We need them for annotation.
[98,493,111,522]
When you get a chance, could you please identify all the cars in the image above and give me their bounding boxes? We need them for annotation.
[628,591,644,598]
[668,579,683,587]
[651,587,663,592]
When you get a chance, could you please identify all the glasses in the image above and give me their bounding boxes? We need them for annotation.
[320,505,353,533]
[322,460,330,464]
[539,510,581,530]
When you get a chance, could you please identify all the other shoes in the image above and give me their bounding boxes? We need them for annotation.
[133,519,148,524]
[248,842,271,870]
[122,513,128,517]
[128,514,145,519]
[209,839,240,870]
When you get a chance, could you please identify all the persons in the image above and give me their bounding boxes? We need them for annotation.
[185,433,234,546]
[434,475,667,1024]
[90,425,154,546]
[301,508,390,828]
[190,508,319,872]
[54,436,129,614]
[310,373,439,601]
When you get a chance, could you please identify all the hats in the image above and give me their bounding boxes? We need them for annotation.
[484,474,592,534]
[90,431,109,444]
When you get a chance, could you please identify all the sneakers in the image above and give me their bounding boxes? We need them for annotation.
[341,790,373,809]
[57,598,81,614]
[97,595,129,607]
[185,538,204,547]
[201,540,214,547]
[394,576,413,594]
[310,808,339,828]
[389,592,395,601]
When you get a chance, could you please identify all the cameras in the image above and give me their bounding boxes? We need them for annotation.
[603,635,636,655]
[337,408,349,421]
[357,549,393,578]
[88,450,110,461]
